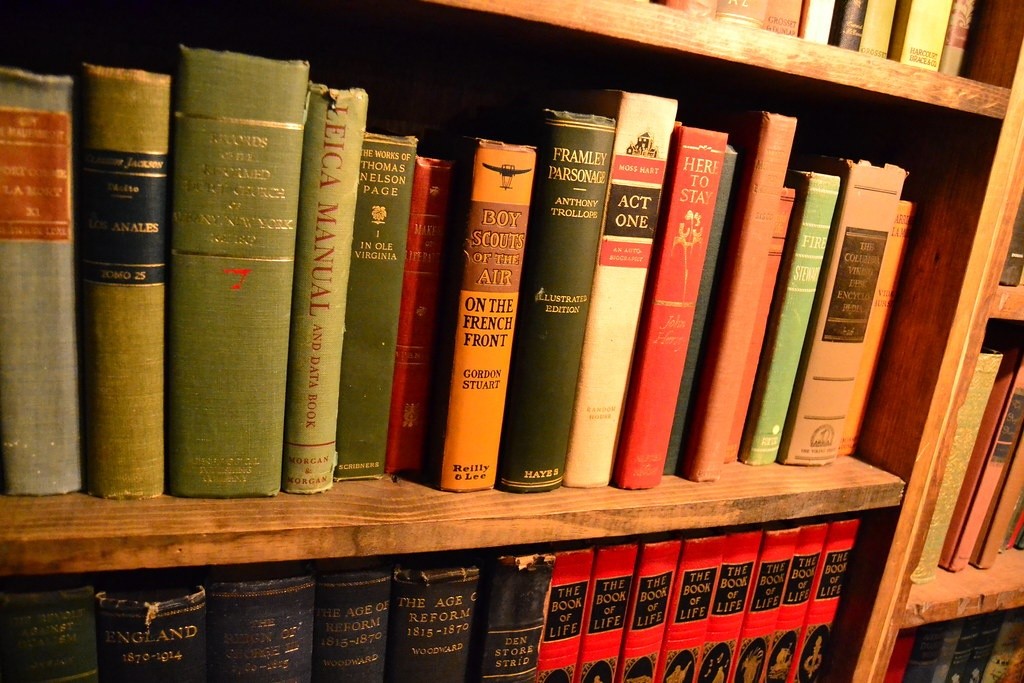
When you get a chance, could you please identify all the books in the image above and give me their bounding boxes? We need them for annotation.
[631,0,975,75]
[0,71,1024,683]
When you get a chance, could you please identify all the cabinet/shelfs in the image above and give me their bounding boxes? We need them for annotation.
[0,0,1024,683]
